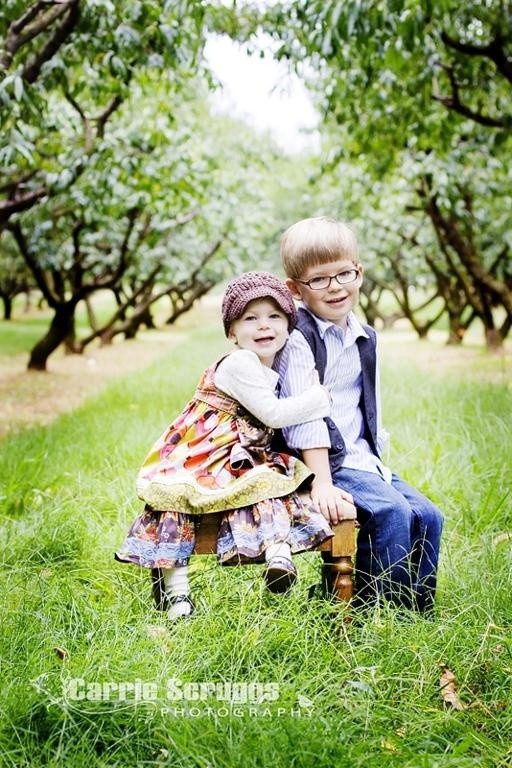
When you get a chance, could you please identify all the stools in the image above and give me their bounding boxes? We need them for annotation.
[144,489,357,635]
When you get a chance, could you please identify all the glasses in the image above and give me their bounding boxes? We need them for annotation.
[294,264,359,290]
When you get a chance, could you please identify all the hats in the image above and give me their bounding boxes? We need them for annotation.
[222,271,298,335]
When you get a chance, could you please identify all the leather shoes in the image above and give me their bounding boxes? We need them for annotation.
[165,594,194,631]
[263,556,297,591]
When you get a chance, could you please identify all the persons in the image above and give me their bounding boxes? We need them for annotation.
[270,213,446,628]
[110,268,334,625]
